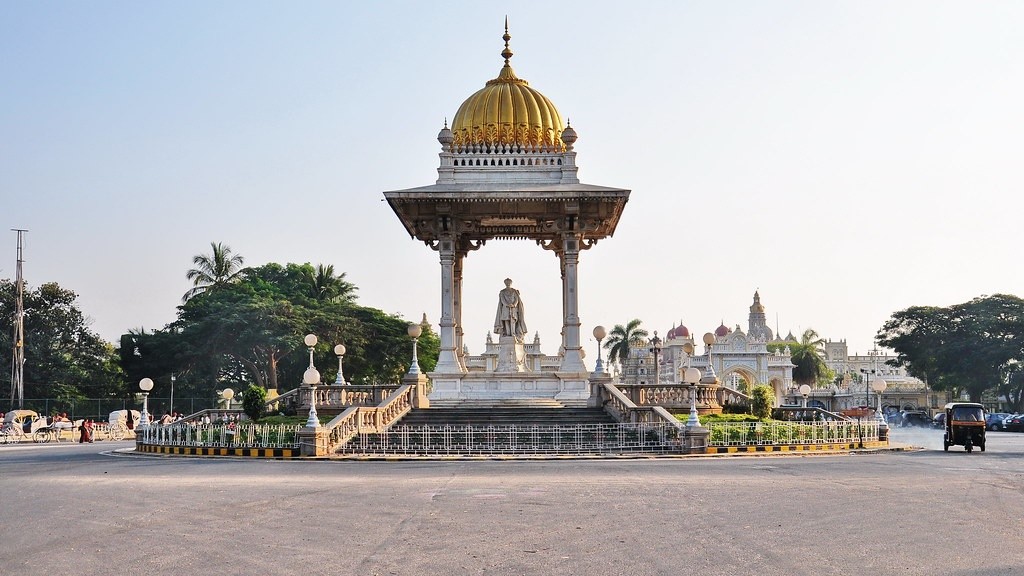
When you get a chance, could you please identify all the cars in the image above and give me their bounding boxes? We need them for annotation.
[932,413,945,430]
[985,413,1024,431]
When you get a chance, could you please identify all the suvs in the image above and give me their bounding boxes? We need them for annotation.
[887,409,932,427]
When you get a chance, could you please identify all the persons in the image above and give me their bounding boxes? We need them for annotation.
[500,278,519,336]
[178,413,183,420]
[82,418,96,443]
[54,413,62,422]
[222,412,239,423]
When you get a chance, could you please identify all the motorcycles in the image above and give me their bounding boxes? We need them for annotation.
[943,403,986,453]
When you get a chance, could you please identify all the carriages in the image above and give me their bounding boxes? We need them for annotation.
[0,410,85,444]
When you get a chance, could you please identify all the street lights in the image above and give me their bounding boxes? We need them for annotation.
[586,326,614,408]
[860,368,876,420]
[304,334,318,369]
[297,369,328,456]
[222,388,234,409]
[330,345,347,405]
[683,343,694,368]
[133,378,154,441]
[873,378,887,422]
[402,325,430,408]
[799,384,812,420]
[699,332,718,402]
[680,367,711,453]
